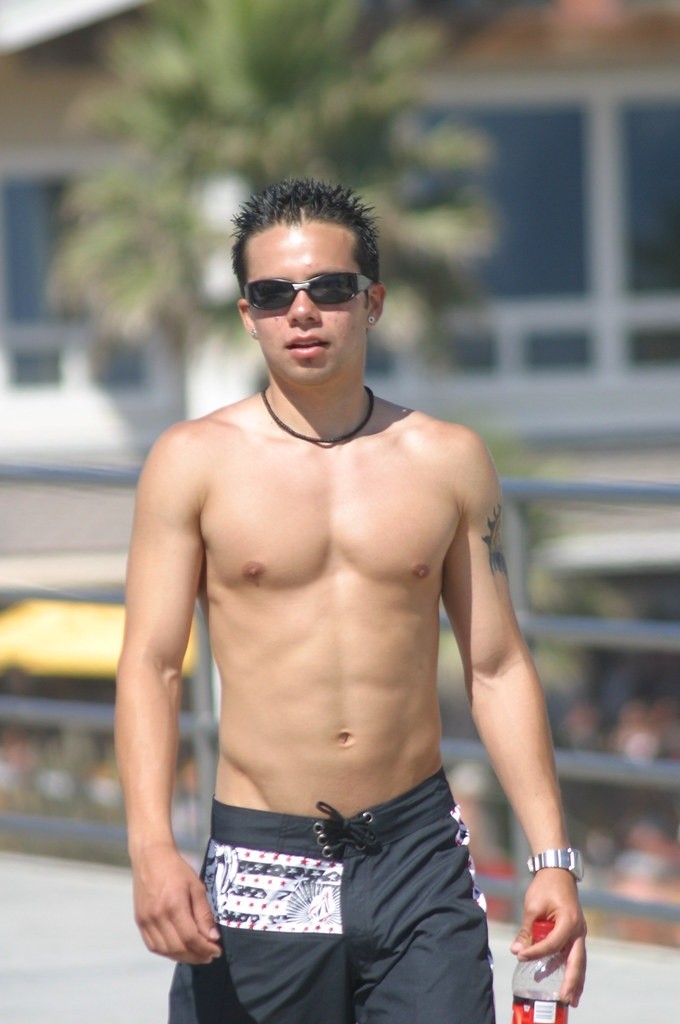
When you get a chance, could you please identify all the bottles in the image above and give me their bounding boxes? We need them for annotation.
[514,921,572,1024]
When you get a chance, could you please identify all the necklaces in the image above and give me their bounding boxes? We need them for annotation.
[261,385,374,443]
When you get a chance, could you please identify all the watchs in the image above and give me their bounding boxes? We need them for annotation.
[527,848,584,882]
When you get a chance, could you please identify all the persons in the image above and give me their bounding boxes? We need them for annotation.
[113,178,588,1024]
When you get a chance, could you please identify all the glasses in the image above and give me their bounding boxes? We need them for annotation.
[244,272,373,310]
